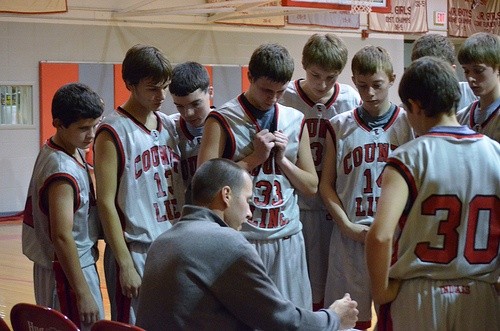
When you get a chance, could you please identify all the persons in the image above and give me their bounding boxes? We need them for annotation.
[318,45,415,331]
[22,83,106,331]
[457,34,500,142]
[195,41,321,311]
[280,31,361,309]
[135,157,358,331]
[94,44,185,327]
[399,30,475,134]
[163,62,218,203]
[367,56,500,330]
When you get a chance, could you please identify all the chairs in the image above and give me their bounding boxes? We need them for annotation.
[0,303,146,331]
